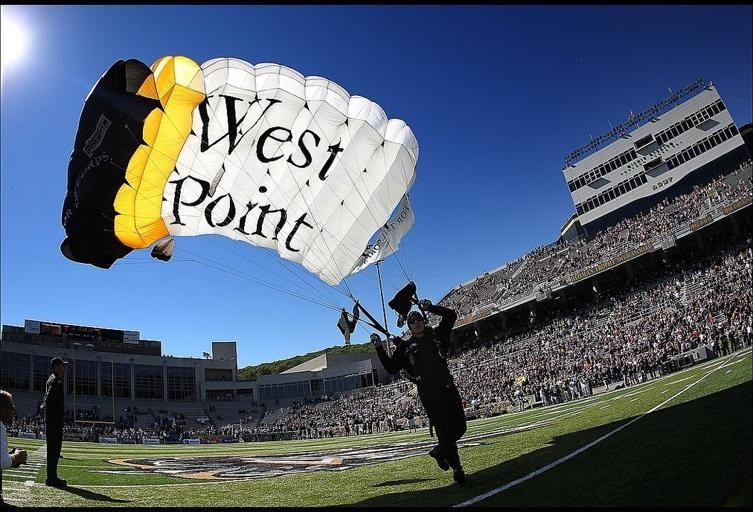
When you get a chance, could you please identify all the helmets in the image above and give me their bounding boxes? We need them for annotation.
[407,311,423,325]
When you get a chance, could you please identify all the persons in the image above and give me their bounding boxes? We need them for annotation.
[36,356,71,486]
[0,390,29,470]
[385,153,752,440]
[369,298,468,483]
[7,380,386,442]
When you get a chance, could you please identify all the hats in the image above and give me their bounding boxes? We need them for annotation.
[51,357,69,366]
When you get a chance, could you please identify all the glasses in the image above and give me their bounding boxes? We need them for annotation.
[409,316,421,325]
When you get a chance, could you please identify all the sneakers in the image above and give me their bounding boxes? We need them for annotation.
[46,476,67,486]
[427,446,449,471]
[453,466,466,480]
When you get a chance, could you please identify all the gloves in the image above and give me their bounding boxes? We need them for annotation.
[419,300,432,312]
[370,333,382,347]
